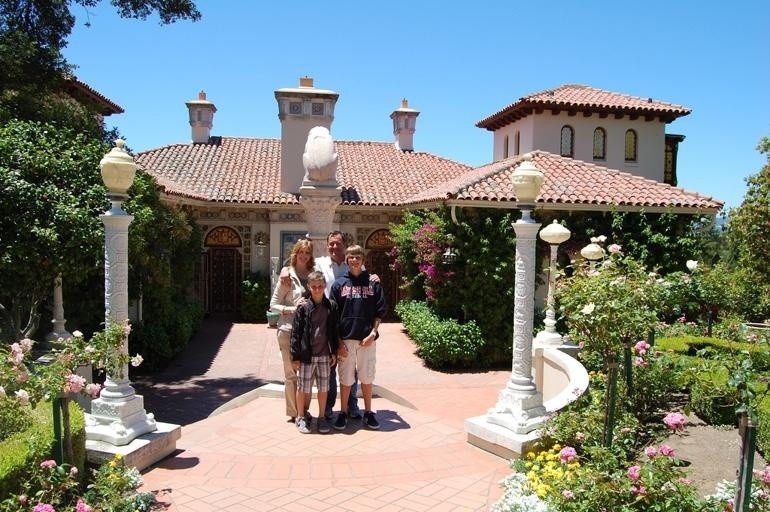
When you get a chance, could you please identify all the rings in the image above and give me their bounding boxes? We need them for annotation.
[343,351,344,353]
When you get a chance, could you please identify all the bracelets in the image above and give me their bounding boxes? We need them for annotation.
[369,328,377,339]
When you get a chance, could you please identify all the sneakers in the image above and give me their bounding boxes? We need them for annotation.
[324,407,335,418]
[362,410,381,430]
[317,417,331,434]
[295,412,313,434]
[333,407,362,431]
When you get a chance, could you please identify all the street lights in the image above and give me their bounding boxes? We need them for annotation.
[484,152,572,436]
[86,137,158,447]
[581,243,606,270]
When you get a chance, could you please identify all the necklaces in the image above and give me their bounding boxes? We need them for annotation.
[299,271,308,278]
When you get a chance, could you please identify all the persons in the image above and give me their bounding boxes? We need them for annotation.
[281,231,381,420]
[270,238,317,424]
[290,270,337,433]
[330,244,386,430]
